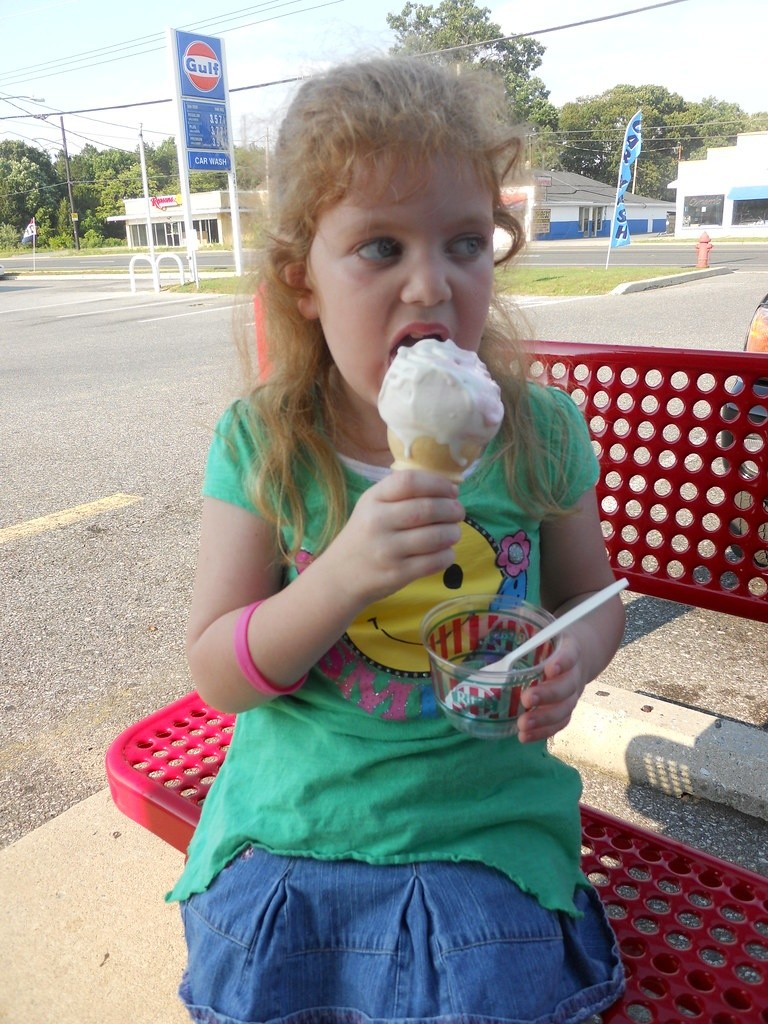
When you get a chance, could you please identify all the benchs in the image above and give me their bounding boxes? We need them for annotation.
[105,337,768,1024]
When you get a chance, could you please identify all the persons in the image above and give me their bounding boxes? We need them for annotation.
[159,53,627,1024]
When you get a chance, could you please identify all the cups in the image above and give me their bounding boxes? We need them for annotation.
[420,594,561,739]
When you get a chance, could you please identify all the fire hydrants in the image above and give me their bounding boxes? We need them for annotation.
[696,231,714,268]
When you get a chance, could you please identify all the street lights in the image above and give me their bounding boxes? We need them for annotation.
[32,137,80,250]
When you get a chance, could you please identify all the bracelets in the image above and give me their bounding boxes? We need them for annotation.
[233,599,310,696]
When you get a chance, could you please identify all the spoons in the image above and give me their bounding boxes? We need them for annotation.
[445,577,633,710]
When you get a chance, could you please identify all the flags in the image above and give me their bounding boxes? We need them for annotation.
[22,219,37,243]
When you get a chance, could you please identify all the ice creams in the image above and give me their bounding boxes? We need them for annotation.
[377,337,505,488]
